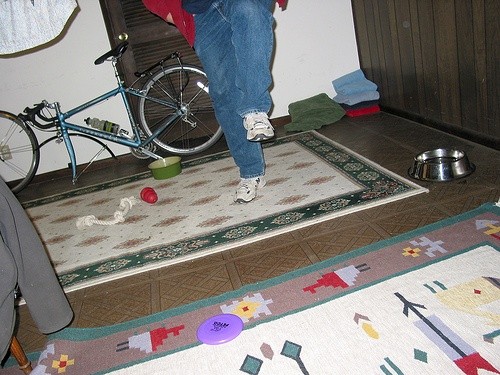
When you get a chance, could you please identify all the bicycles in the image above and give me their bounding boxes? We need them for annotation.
[0,40,224,195]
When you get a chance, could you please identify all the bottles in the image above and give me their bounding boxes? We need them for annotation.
[84,117,119,135]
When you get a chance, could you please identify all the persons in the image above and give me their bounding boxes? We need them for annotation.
[142,0,286,204]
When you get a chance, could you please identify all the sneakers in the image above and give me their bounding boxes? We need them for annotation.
[233,176,267,203]
[241,111,275,142]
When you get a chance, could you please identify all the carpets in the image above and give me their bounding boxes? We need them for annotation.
[14,129,430,306]
[0,203,500,375]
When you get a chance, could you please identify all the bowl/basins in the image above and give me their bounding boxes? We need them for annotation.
[148,156,183,180]
[408,148,476,183]
